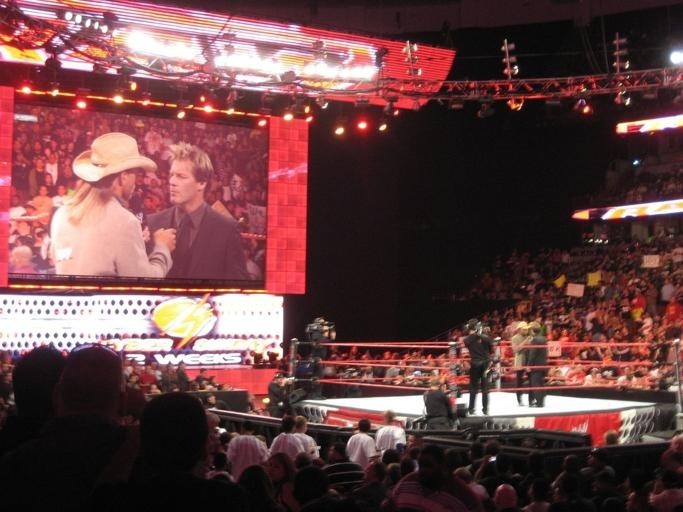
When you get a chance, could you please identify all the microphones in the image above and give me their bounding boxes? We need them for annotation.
[128,189,151,254]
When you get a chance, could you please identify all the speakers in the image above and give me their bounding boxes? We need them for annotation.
[217,389,248,435]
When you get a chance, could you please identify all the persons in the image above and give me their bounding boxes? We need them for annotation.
[11,103,270,281]
[323,144,683,416]
[1,341,682,512]
[267,318,336,417]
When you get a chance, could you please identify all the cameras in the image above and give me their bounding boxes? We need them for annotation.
[488,455,498,463]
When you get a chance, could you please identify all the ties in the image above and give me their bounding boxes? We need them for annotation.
[172,215,192,262]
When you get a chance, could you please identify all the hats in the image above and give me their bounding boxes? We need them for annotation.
[72,132,158,182]
